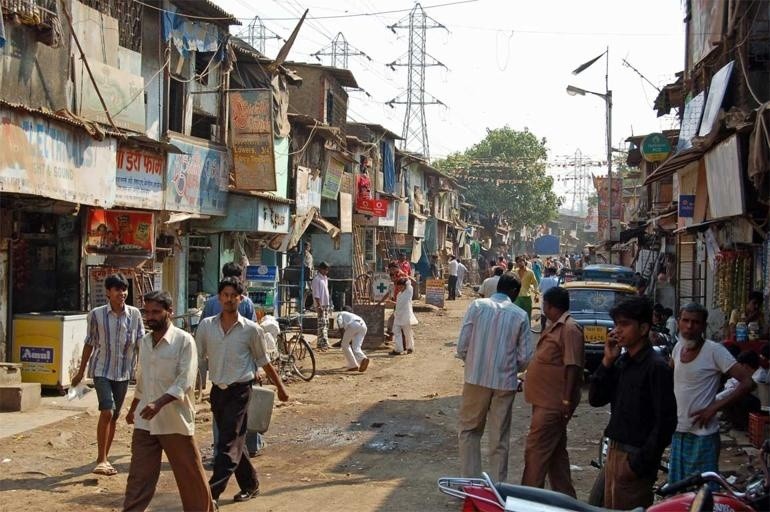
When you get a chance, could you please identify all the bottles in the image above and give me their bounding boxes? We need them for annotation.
[736,317,748,343]
[748,322,760,341]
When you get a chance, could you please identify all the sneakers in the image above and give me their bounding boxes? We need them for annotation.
[389,350,401,355]
[359,358,369,372]
[407,348,412,354]
[348,367,359,371]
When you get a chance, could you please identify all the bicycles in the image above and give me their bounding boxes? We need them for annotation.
[267,312,317,385]
[206,309,207,402]
[529,306,544,336]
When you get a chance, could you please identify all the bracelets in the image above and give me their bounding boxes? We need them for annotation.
[561,399,576,406]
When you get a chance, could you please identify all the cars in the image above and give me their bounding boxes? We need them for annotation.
[554,253,642,382]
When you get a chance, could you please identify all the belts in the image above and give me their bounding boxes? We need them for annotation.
[608,438,636,453]
[211,379,252,391]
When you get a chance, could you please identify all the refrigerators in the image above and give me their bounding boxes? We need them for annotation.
[11,312,95,392]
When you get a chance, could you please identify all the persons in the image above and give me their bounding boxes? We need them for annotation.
[454,271,533,492]
[69,273,146,476]
[517,285,586,502]
[632,272,770,433]
[120,291,218,512]
[667,301,760,499]
[310,260,334,351]
[193,276,291,506]
[373,251,590,357]
[302,241,314,270]
[336,305,368,373]
[195,261,269,458]
[585,292,675,512]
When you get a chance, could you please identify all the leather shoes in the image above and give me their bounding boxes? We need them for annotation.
[232,484,262,502]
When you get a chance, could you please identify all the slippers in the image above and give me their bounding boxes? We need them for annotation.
[91,459,118,475]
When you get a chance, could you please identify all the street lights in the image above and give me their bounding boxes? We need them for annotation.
[565,84,616,265]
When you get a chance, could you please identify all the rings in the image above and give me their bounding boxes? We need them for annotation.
[565,415,568,418]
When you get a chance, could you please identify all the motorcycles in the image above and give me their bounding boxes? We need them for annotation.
[436,460,770,511]
